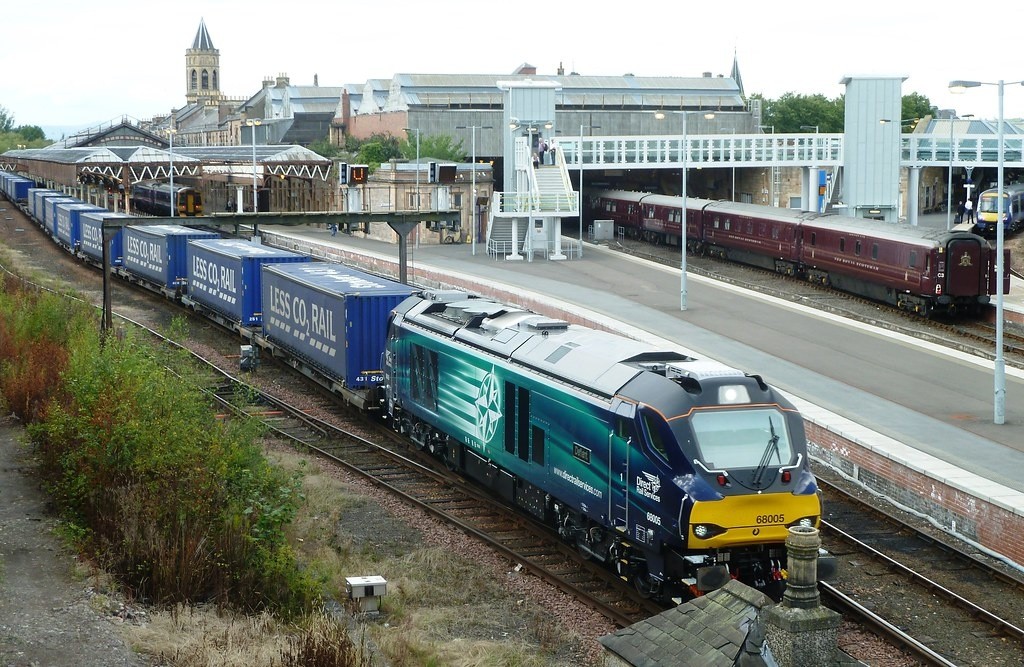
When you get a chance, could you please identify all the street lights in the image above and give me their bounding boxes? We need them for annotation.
[456,126,494,256]
[948,80,1023,423]
[165,126,177,219]
[576,125,603,259]
[400,127,420,249]
[247,118,262,212]
[653,111,716,312]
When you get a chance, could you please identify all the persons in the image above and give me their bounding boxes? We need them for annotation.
[538,138,545,164]
[965,199,975,223]
[957,201,965,223]
[532,153,539,169]
[550,139,556,165]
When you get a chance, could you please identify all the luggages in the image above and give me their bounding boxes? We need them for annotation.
[954,215,960,223]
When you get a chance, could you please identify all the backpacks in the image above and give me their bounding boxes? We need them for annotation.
[544,144,548,151]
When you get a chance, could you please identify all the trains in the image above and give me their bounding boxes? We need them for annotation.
[134,180,203,220]
[575,187,1012,321]
[0,170,822,611]
[976,183,1024,238]
